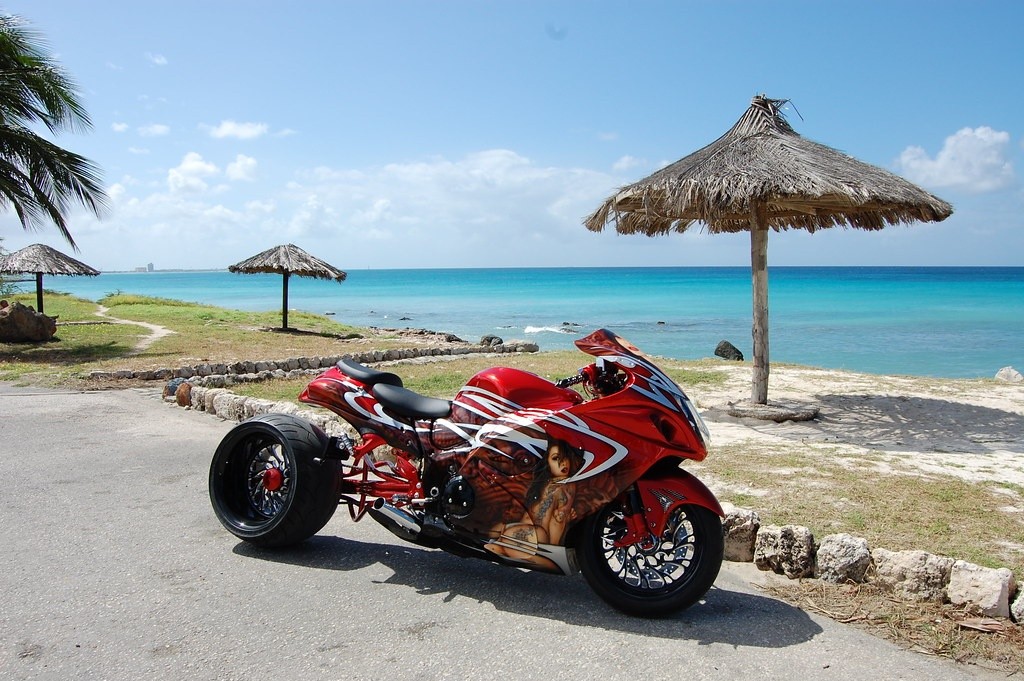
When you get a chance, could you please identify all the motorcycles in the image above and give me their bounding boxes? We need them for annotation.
[209,328,727,616]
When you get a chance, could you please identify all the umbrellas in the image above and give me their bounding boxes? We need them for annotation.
[0,244,101,313]
[227,243,347,327]
[581,90,953,405]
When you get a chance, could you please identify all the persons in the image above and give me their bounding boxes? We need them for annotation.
[484,438,577,573]
